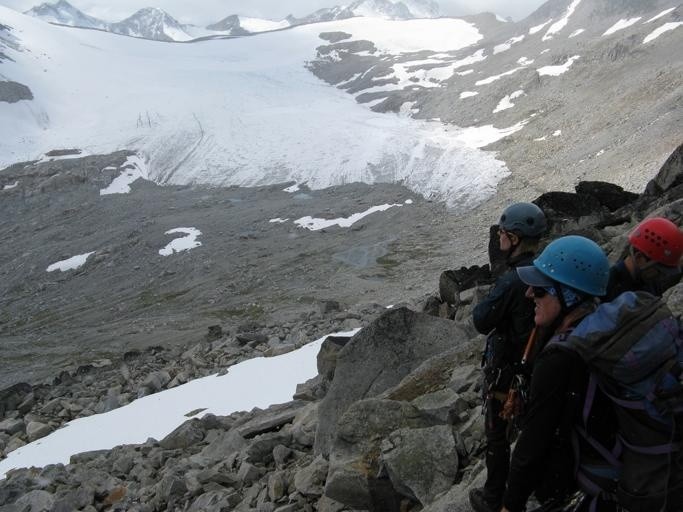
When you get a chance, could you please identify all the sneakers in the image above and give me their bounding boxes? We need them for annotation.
[469,488,493,512]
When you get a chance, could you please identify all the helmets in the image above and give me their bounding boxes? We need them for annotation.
[628,217,683,267]
[533,235,610,296]
[498,202,547,237]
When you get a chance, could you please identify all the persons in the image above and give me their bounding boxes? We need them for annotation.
[468,200,547,512]
[498,234,682,512]
[603,217,683,305]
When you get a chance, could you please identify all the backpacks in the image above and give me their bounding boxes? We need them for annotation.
[546,291,682,512]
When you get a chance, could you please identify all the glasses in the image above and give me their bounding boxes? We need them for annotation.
[532,287,548,297]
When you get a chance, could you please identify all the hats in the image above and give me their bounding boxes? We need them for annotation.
[515,265,554,286]
[655,265,682,275]
